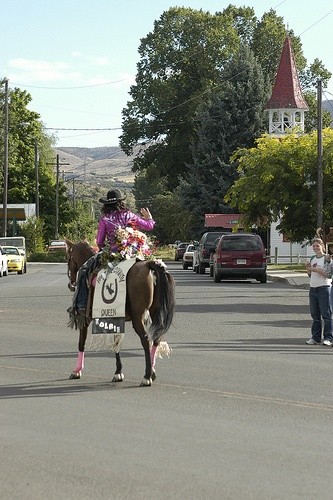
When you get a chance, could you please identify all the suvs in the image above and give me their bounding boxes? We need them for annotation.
[0,237,27,273]
[194,232,227,275]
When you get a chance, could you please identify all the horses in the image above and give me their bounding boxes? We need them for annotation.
[63,235,175,387]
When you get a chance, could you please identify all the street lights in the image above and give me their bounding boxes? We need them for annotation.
[59,172,96,185]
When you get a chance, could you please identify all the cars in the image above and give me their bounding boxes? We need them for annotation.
[0,246,26,278]
[175,233,266,283]
[46,240,70,252]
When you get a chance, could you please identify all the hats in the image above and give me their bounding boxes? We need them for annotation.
[98,187,127,203]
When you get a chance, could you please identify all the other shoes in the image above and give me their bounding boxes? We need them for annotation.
[79,305,86,311]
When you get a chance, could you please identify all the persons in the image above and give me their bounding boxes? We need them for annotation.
[305,238,333,346]
[73,188,155,310]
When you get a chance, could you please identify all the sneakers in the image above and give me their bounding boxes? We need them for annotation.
[322,340,333,346]
[305,339,320,345]
